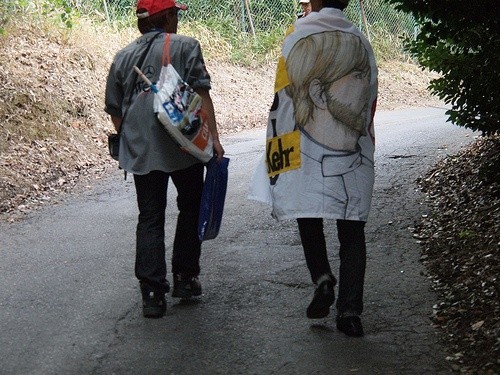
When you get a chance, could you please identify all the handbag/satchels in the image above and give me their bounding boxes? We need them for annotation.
[198,157,229,242]
[108,134,120,161]
[154,33,212,163]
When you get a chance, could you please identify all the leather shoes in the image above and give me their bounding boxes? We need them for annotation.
[141,290,166,318]
[336,314,364,337]
[305,280,335,319]
[172,275,202,298]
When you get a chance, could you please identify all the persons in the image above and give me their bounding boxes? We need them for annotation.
[266,0,378,338]
[104,0,225,318]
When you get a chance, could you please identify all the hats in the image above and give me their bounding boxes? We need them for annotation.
[299,0,309,4]
[135,0,187,17]
